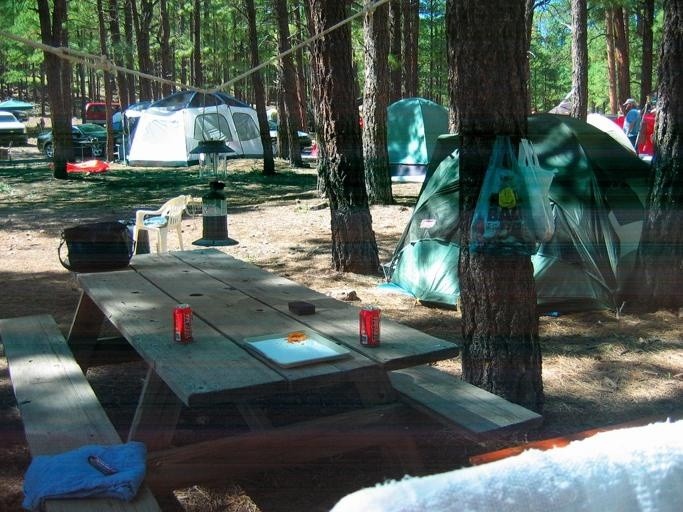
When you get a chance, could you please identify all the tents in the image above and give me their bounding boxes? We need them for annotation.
[549,108,638,157]
[390,114,655,313]
[386,96,450,181]
[612,114,655,157]
[127,89,277,167]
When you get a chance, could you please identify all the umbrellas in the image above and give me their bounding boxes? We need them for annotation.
[0,97,35,109]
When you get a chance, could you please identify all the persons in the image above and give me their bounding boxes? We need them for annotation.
[622,98,641,147]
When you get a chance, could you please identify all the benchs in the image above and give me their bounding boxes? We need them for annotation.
[0,313,160,511]
[384,363,546,446]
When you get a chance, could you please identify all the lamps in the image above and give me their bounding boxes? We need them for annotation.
[187,87,239,248]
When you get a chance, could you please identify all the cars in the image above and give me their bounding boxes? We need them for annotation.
[84,102,120,122]
[266,121,312,154]
[0,108,27,144]
[36,123,115,161]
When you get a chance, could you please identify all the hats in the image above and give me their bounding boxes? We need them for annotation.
[623,99,636,106]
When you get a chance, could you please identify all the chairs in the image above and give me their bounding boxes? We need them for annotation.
[129,195,188,257]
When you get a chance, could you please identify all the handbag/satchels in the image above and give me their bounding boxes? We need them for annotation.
[58,222,135,272]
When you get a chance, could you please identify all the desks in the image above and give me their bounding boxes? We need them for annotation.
[62,248,459,486]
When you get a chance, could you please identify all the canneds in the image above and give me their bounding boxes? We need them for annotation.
[173,303,192,343]
[359,306,381,347]
